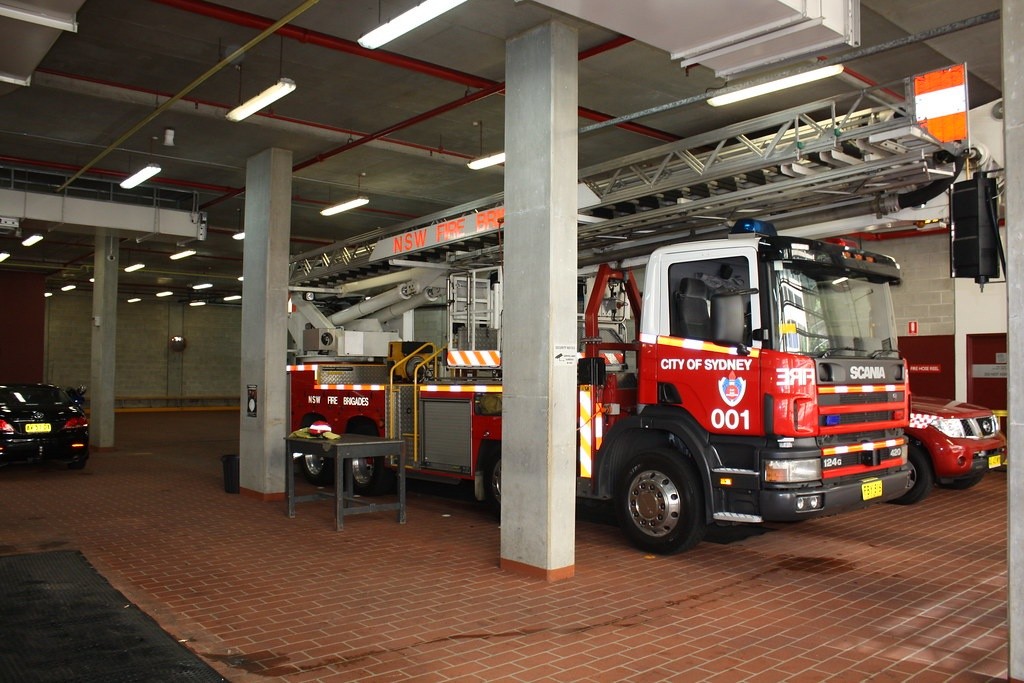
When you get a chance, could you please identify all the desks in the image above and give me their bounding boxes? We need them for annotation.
[283,434,406,532]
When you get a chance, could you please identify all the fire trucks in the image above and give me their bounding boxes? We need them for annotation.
[285,218,917,556]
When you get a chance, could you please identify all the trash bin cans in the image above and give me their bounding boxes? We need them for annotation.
[220,453,240,493]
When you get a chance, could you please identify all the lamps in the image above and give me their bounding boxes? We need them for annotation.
[708,63,845,107]
[319,175,369,216]
[169,236,196,261]
[356,0,467,50]
[118,139,162,190]
[466,121,506,170]
[224,34,296,123]
[232,199,245,240]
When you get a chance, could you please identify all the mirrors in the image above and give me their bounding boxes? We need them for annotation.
[171,335,187,352]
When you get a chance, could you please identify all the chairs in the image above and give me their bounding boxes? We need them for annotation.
[674,277,712,340]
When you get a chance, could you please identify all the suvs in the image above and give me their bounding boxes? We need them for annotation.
[884,394,1007,507]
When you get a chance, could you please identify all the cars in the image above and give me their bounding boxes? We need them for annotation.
[0,385,90,470]
